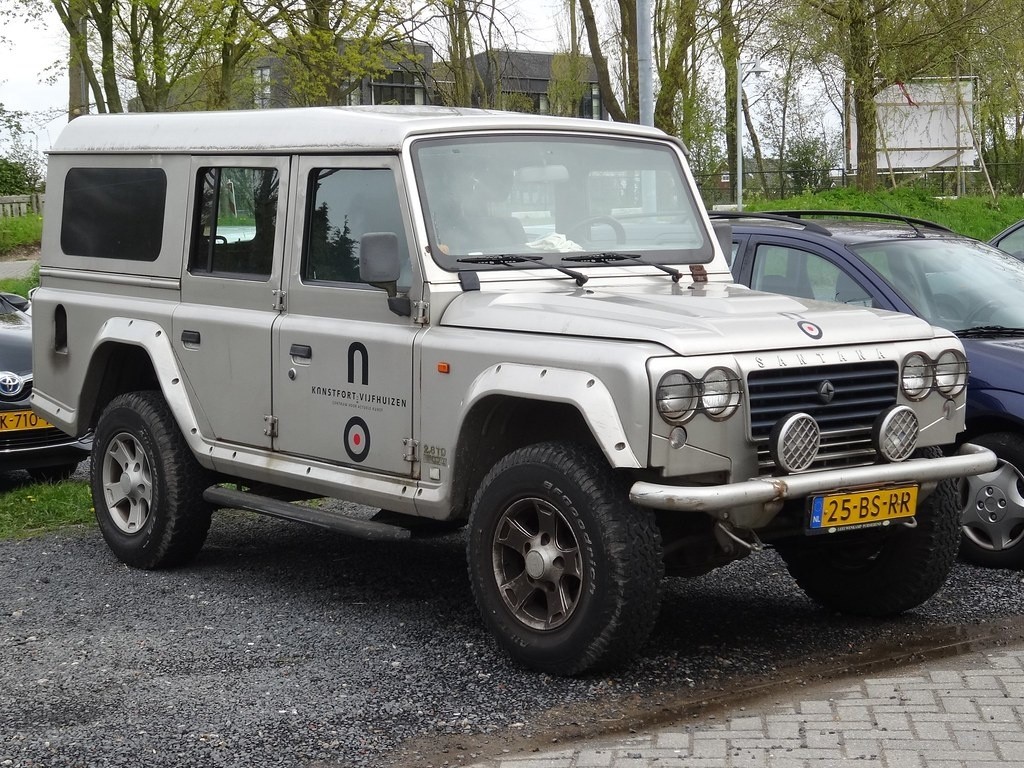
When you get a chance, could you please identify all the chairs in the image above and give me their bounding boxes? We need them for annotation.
[835,270,872,304]
[471,166,528,249]
[761,274,795,294]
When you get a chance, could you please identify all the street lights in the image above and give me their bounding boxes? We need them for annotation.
[736,58,770,212]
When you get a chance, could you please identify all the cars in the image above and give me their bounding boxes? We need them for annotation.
[0,293,94,482]
[925,219,1024,321]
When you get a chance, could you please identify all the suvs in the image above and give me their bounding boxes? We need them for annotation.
[29,104,998,685]
[527,210,1024,568]
